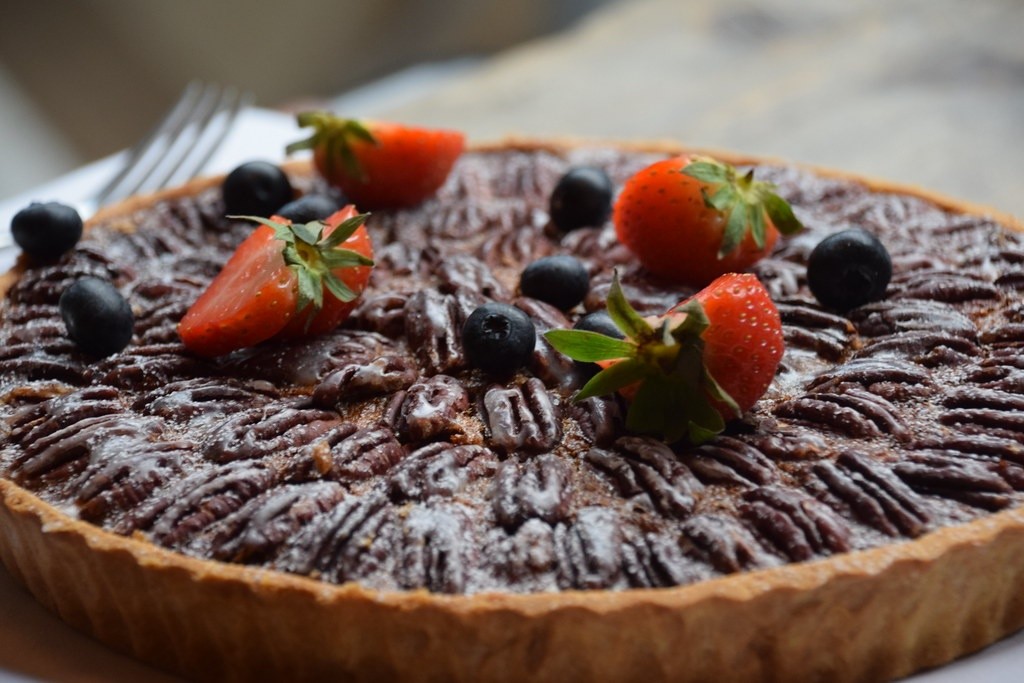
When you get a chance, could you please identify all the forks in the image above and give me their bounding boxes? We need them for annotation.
[88,77,253,215]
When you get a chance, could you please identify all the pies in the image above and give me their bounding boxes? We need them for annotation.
[0,107,1024,683]
